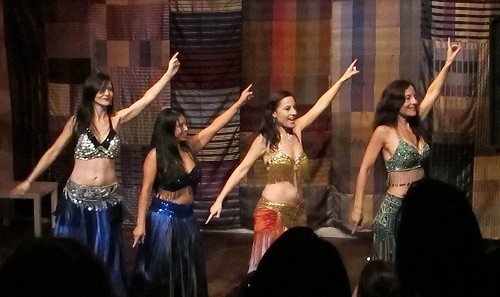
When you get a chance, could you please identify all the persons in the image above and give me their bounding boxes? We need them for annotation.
[204,59,359,275]
[349,37,461,264]
[132,83,253,297]
[0,181,500,297]
[10,51,180,297]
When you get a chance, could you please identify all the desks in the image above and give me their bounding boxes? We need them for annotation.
[0,180,58,238]
